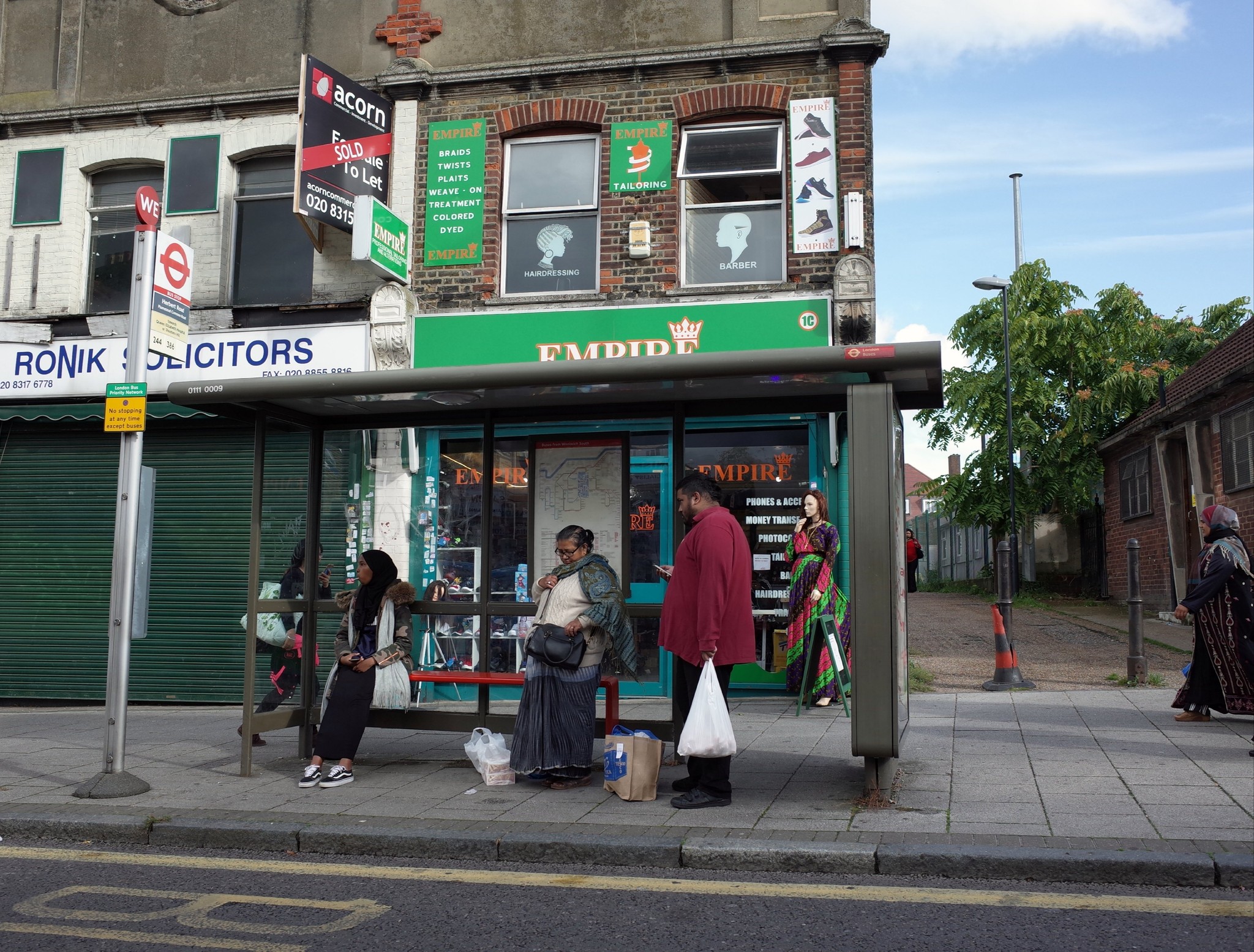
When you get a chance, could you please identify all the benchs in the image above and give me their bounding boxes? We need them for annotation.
[410,671,619,734]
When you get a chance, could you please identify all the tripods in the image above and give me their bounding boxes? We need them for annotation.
[413,614,462,708]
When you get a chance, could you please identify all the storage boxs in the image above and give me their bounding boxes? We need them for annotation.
[515,564,532,602]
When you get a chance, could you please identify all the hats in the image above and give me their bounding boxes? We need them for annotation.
[1201,505,1219,528]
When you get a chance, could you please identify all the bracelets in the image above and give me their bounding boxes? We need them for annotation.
[286,635,296,640]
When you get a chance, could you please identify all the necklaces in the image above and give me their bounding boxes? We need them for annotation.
[804,523,821,550]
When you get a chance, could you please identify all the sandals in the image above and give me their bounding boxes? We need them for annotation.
[551,773,592,789]
[541,776,568,786]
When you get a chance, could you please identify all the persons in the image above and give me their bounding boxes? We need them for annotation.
[238,538,331,747]
[906,528,921,593]
[299,550,417,788]
[1171,505,1254,722]
[420,580,454,620]
[1249,735,1254,757]
[510,525,638,789]
[657,471,758,810]
[784,489,852,706]
[518,616,530,637]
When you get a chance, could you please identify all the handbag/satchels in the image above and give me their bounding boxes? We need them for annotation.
[463,727,515,786]
[677,656,737,758]
[603,725,666,801]
[526,623,586,671]
[913,539,924,559]
[240,568,304,647]
[1182,662,1191,678]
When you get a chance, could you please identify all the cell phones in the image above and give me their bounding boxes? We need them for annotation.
[319,564,334,580]
[653,565,672,577]
[348,658,360,662]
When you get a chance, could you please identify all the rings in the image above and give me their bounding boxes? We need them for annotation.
[570,627,574,629]
[548,581,551,584]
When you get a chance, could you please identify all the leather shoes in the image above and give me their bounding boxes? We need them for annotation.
[672,775,695,792]
[670,787,732,809]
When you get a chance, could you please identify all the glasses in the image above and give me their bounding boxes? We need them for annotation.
[555,542,586,557]
[318,552,323,558]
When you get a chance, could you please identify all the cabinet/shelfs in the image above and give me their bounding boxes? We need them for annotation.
[435,547,525,673]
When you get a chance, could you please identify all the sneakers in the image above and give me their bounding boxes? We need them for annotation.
[432,516,531,674]
[298,764,322,787]
[318,765,354,787]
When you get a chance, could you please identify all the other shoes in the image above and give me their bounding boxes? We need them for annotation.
[1249,750,1254,757]
[237,724,267,746]
[1174,710,1210,722]
